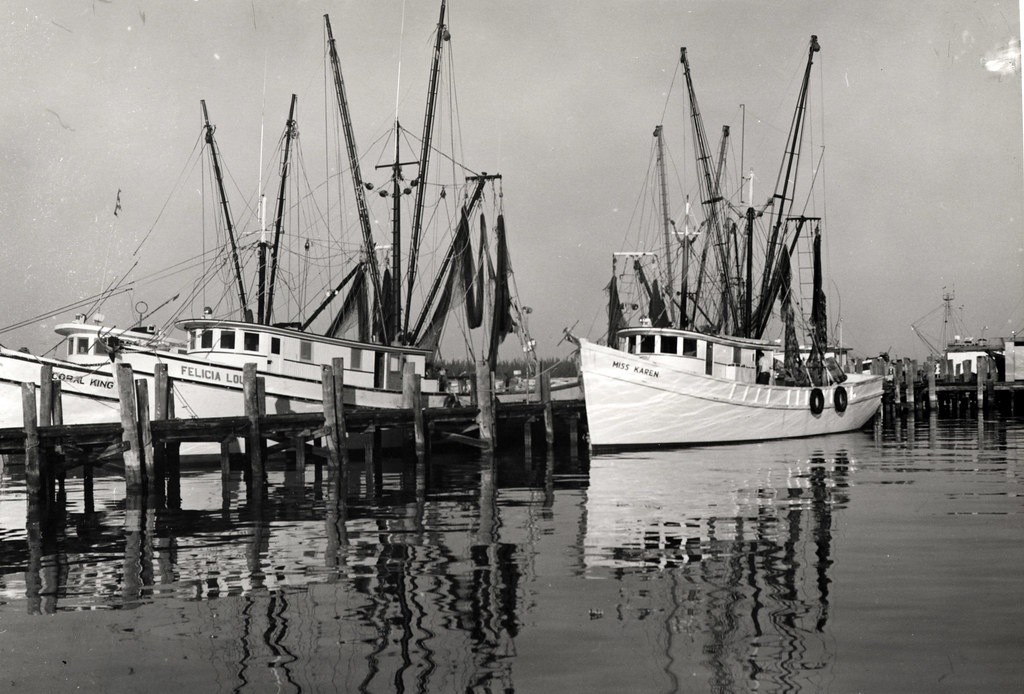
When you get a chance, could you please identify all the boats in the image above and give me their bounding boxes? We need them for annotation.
[0,0,584,472]
[576,34,885,446]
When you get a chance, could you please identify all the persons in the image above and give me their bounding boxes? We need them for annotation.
[756,352,771,384]
